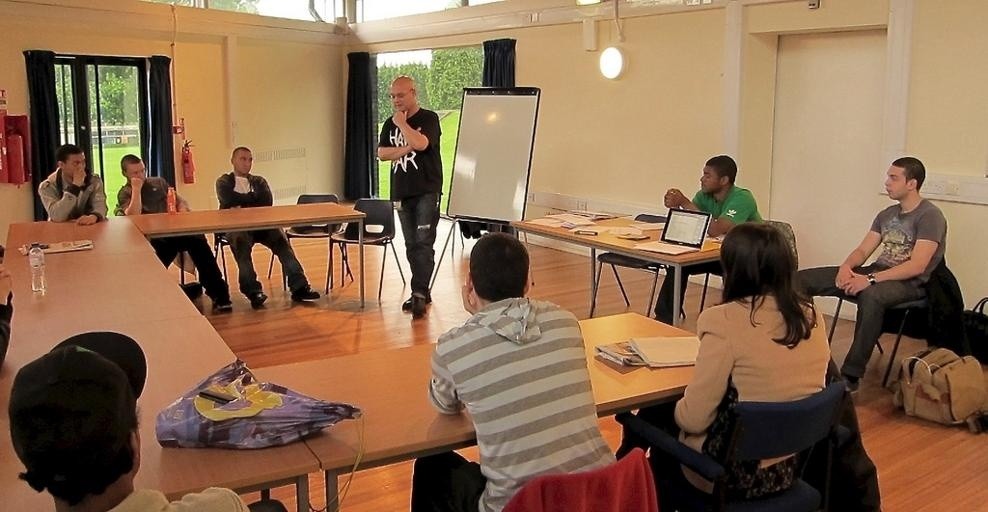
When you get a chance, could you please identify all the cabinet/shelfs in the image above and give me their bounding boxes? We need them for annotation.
[167,187,175,215]
[30,243,48,291]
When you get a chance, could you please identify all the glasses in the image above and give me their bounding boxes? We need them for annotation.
[598,46,628,80]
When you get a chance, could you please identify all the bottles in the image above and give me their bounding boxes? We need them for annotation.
[9,332,145,493]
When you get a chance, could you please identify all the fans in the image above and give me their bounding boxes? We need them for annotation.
[446,86,541,227]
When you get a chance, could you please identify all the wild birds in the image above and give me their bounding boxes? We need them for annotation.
[633,207,712,255]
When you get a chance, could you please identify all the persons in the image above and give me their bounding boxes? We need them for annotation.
[38,144,108,226]
[410,233,616,511]
[215,147,321,310]
[377,77,443,321]
[9,331,290,512]
[797,157,948,396]
[652,154,764,329]
[615,223,831,506]
[116,154,233,311]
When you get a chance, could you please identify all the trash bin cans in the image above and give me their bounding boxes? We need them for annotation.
[866,273,876,286]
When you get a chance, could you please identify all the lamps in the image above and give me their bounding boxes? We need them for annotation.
[598,46,628,80]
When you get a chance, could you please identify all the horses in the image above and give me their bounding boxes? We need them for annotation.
[199,390,239,405]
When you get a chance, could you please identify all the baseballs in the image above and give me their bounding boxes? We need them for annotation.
[4,125,27,184]
[181,139,194,184]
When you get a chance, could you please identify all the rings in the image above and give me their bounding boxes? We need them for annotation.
[575,231,599,235]
[76,243,94,248]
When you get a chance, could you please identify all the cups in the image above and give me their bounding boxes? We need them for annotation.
[390,90,412,99]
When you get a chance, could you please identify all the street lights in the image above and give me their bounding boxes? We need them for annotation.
[962,310,988,364]
[902,347,988,426]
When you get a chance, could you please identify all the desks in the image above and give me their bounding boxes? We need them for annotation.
[248,312,701,512]
[510,210,728,329]
[1,215,321,511]
[123,202,366,306]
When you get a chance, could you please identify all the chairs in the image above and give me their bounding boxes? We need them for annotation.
[444,379,851,512]
[215,297,232,310]
[252,292,266,306]
[292,290,320,302]
[589,215,945,389]
[403,292,431,316]
[0,185,408,307]
